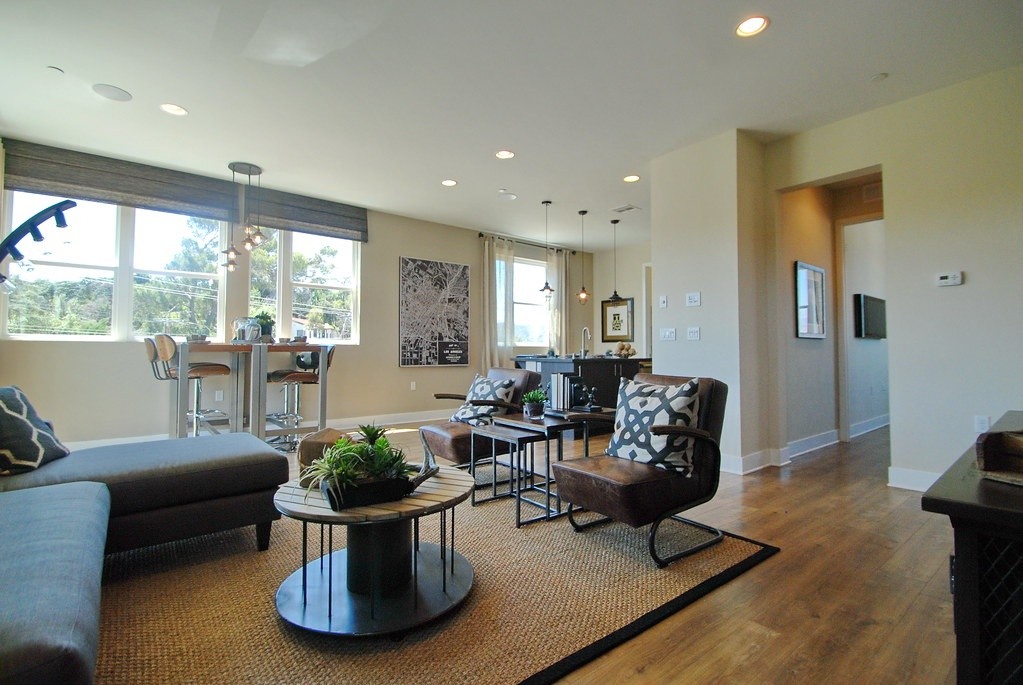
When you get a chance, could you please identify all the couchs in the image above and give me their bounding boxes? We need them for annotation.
[0,386,290,685]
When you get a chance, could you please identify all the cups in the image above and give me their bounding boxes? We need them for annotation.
[187,334,206,341]
[294,336,307,342]
[279,338,290,344]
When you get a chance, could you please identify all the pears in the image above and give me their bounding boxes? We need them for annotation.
[613,342,635,354]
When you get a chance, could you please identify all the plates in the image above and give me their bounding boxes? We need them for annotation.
[289,342,309,346]
[188,341,210,344]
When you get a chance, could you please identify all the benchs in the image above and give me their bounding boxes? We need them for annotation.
[469,426,562,529]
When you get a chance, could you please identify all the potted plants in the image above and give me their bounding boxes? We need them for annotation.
[522,389,551,420]
[289,419,419,513]
[255,311,276,342]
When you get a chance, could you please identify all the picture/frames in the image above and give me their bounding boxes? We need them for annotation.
[601,297,634,343]
[399,256,471,367]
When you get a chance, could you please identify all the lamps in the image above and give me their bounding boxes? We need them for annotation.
[575,211,590,306]
[221,162,268,272]
[608,219,622,308]
[539,201,555,296]
[0,273,17,294]
[54,208,75,246]
[28,222,56,259]
[5,244,41,277]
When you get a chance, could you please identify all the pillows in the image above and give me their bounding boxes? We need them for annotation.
[0,385,70,476]
[448,373,517,428]
[603,377,701,479]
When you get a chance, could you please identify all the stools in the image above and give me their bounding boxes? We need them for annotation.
[267,352,318,445]
[155,334,230,437]
[144,338,222,435]
[270,345,336,454]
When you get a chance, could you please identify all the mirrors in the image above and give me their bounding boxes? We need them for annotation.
[794,260,826,339]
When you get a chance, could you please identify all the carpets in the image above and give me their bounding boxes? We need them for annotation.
[96,457,781,685]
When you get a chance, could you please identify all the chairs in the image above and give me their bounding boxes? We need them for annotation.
[419,368,542,489]
[552,373,728,568]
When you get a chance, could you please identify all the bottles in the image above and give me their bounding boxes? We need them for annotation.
[245,319,261,340]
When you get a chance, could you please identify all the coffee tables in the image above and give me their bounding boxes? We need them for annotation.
[274,463,476,645]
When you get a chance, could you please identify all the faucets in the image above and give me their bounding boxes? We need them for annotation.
[581,327,591,357]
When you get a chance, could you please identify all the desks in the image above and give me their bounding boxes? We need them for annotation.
[174,342,327,451]
[492,408,617,522]
[922,410,1023,685]
